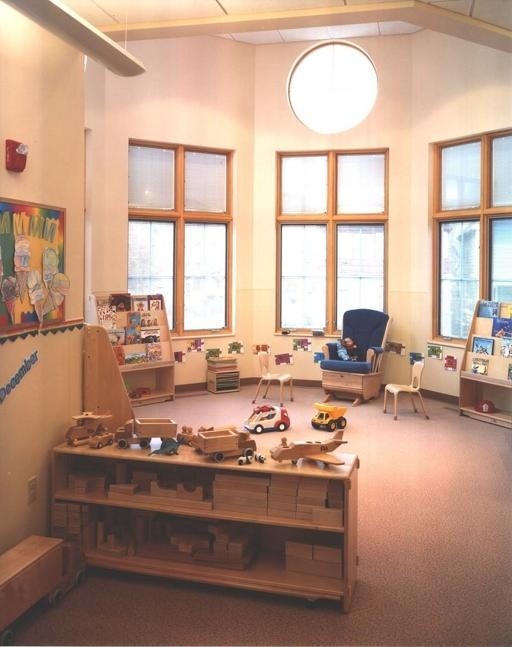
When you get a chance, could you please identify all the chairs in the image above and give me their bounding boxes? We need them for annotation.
[383,361,430,420]
[252,351,293,407]
[319,308,394,407]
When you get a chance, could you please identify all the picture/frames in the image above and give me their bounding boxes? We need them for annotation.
[0,196,67,336]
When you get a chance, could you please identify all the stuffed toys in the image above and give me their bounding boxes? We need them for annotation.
[343,337,360,361]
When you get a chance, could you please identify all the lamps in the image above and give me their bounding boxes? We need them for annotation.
[0,0,147,77]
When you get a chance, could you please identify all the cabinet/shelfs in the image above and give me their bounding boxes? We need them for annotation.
[207,369,241,394]
[89,294,175,407]
[458,299,512,429]
[51,439,360,614]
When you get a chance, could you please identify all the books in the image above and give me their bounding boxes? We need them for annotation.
[207,357,239,390]
[469,300,512,384]
[96,293,162,368]
